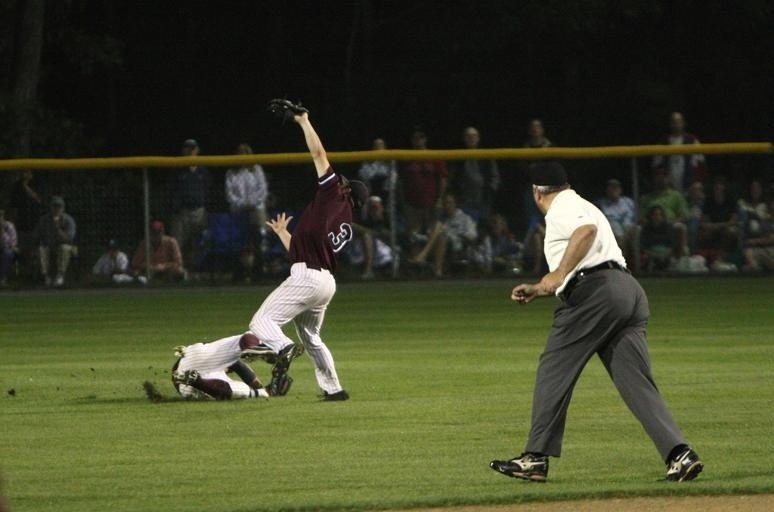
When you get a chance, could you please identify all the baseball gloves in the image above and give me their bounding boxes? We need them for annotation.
[267,98,309,124]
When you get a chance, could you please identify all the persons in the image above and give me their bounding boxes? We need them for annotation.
[172,332,293,402]
[248,99,369,401]
[490,161,704,482]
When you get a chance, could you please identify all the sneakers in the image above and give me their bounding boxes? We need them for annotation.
[269,342,305,380]
[313,390,349,402]
[656,448,704,484]
[488,449,553,485]
[166,370,199,386]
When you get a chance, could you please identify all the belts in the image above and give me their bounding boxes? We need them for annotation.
[561,260,633,300]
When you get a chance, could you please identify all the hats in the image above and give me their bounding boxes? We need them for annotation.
[522,162,570,187]
[148,219,166,232]
[105,236,122,250]
[183,137,199,147]
[341,171,371,204]
[49,195,67,208]
